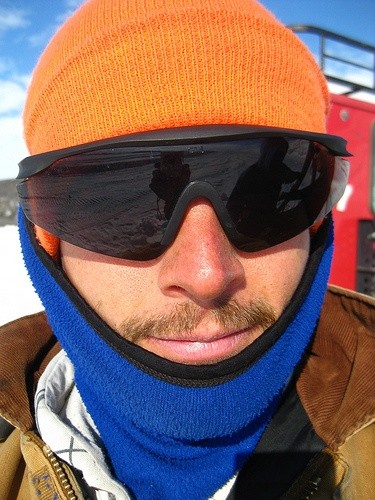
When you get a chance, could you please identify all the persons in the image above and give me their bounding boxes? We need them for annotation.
[227,136,299,240]
[1,1,375,499]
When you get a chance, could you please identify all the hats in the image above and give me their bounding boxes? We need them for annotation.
[23,0,331,261]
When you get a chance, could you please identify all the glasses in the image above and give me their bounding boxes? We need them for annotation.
[16,124,354,261]
